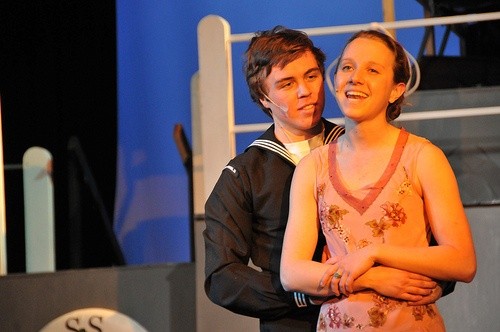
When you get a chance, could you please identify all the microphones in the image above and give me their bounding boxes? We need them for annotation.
[334,83,339,95]
[260,88,288,118]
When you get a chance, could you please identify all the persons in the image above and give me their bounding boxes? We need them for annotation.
[203,23,456,332]
[280,30,477,332]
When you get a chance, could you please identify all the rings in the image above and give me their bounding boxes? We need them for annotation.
[334,272,342,279]
